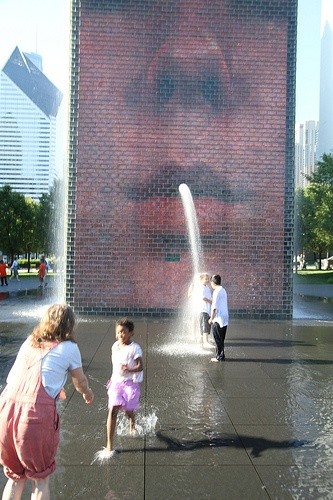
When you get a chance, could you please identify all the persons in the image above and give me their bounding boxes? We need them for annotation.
[0,256,56,287]
[106,319,146,452]
[294,251,320,270]
[189,273,229,362]
[0,304,94,500]
